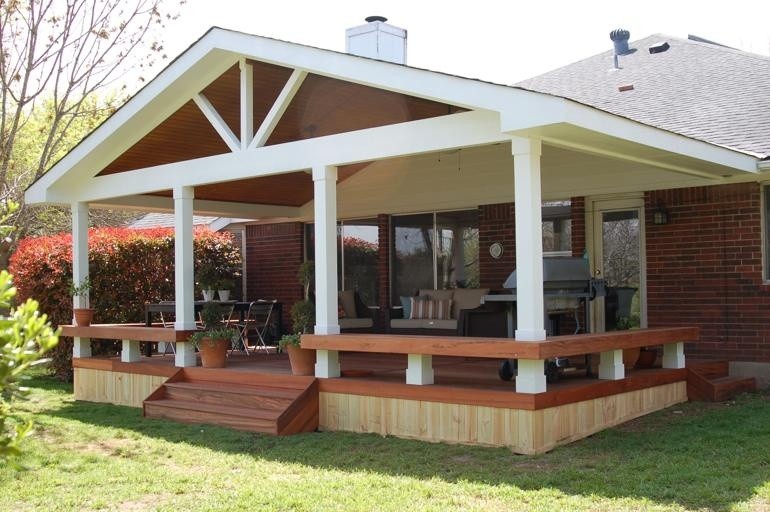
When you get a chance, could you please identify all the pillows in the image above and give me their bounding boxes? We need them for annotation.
[409,296,450,320]
[450,287,491,320]
[400,296,411,319]
[338,299,346,318]
[419,288,455,300]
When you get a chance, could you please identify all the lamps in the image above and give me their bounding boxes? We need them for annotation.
[653,199,670,226]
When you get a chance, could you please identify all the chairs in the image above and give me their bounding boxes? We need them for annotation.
[338,290,374,334]
[159,298,278,360]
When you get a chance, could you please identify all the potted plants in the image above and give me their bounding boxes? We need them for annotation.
[190,328,234,368]
[200,264,216,301]
[217,281,231,302]
[279,333,315,376]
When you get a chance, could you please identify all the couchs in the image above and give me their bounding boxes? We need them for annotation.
[390,287,490,336]
